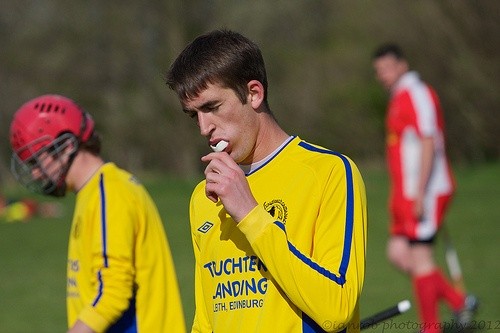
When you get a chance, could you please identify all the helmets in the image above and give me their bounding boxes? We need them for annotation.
[10,95,94,162]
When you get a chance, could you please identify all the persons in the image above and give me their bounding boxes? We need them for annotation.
[9,95,187,333]
[165,27,368,333]
[370,41,479,333]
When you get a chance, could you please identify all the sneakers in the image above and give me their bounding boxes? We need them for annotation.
[455,295,477,332]
